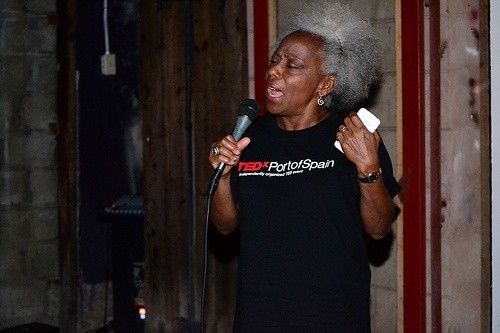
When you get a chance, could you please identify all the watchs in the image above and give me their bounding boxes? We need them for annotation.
[359,168,382,184]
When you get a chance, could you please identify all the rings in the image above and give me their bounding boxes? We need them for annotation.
[213,146,220,155]
[336,134,342,138]
[340,126,346,132]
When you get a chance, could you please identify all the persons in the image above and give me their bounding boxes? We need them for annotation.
[208,3,401,333]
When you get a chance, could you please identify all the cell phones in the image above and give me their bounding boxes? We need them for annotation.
[334,108,381,153]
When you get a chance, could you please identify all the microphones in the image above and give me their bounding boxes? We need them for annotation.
[208,98,260,192]
